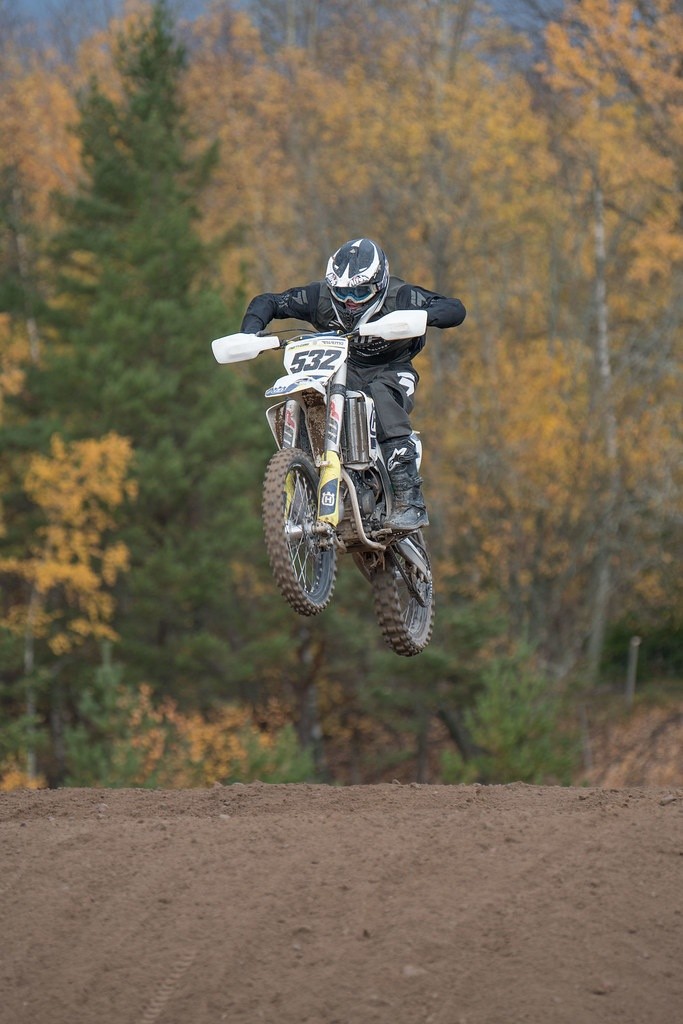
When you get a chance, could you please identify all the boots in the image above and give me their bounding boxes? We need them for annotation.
[382,437,430,530]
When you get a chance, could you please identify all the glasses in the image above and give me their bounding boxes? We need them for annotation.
[326,270,389,304]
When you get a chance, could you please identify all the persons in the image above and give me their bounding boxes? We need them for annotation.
[239,237,468,531]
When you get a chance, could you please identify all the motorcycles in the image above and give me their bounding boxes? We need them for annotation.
[208,305,438,658]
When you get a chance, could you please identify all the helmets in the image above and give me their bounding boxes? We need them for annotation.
[324,238,391,332]
